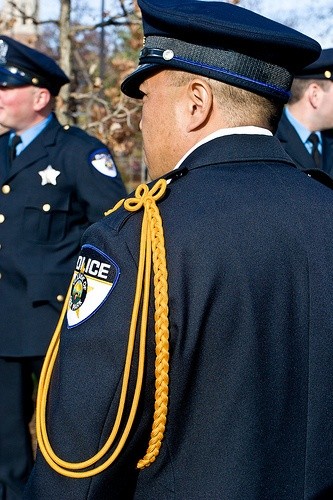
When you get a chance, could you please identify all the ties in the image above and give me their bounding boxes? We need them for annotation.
[9,135,20,162]
[308,133,322,169]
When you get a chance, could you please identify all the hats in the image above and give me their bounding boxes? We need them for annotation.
[121,0,322,105]
[298,46,333,81]
[0,35,70,96]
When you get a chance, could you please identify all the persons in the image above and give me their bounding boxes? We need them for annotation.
[0,30,131,500]
[267,45,333,196]
[19,1,333,500]
